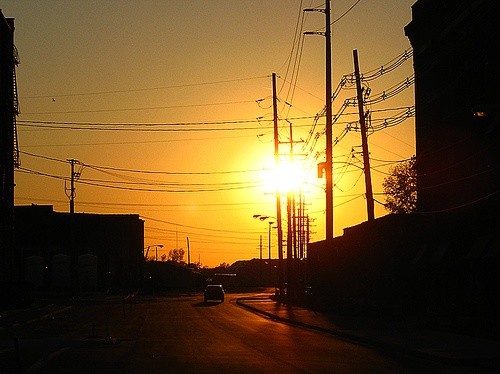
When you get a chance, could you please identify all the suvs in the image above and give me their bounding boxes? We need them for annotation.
[203,284,225,302]
